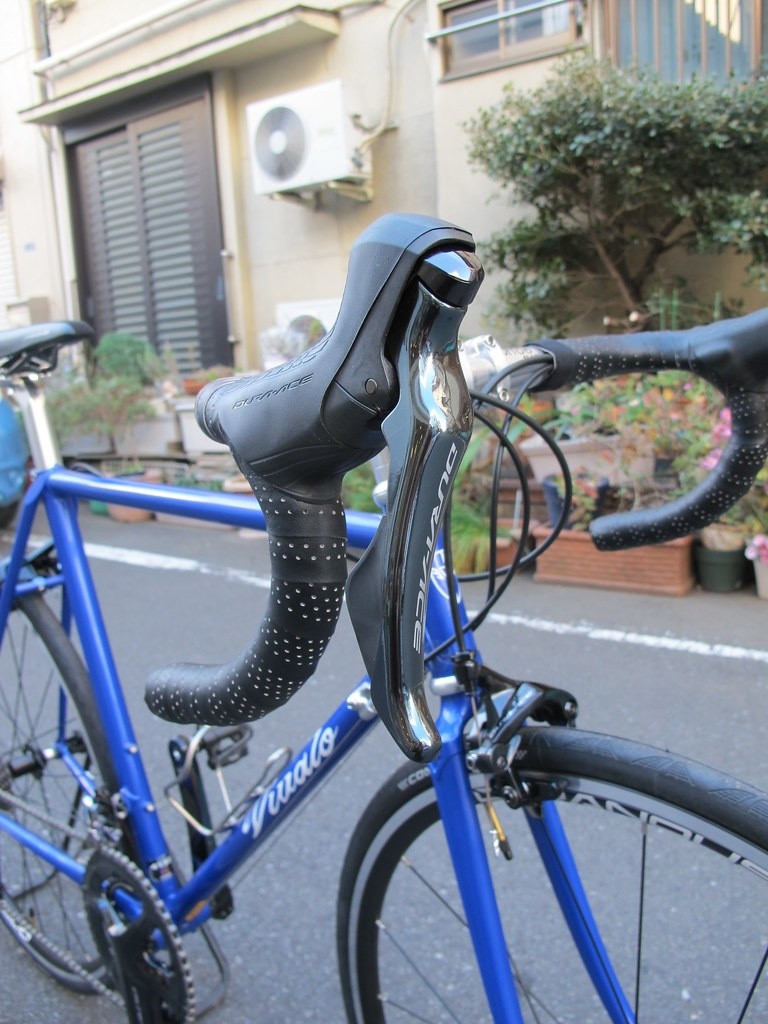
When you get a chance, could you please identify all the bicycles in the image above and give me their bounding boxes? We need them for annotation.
[1,214,768,1024]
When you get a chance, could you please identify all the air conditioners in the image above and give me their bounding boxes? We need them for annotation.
[240,80,378,217]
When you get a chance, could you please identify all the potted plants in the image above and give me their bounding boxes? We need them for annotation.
[53,329,768,601]
